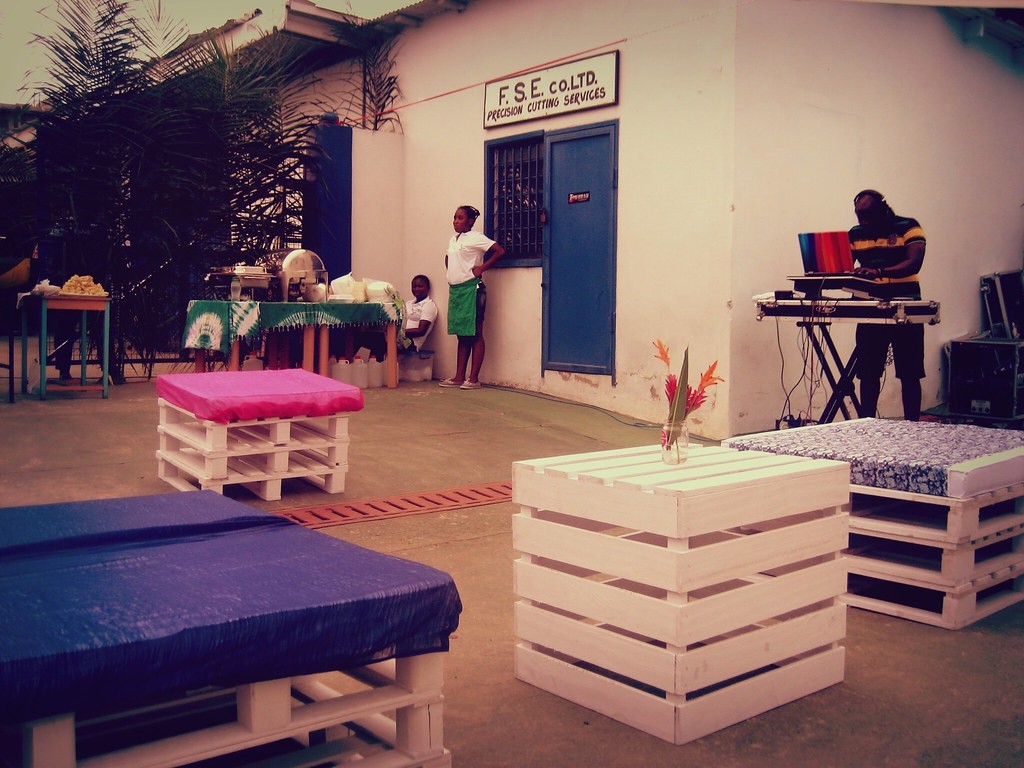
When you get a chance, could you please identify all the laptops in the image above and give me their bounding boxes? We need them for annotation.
[798,232,875,279]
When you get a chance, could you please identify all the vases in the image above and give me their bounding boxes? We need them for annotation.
[662,422,689,464]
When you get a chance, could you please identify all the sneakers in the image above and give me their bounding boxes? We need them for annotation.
[439,378,481,389]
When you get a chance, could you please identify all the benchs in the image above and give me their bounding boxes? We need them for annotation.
[0,490,294,557]
[0,523,465,768]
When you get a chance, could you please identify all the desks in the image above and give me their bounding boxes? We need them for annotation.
[20,291,112,398]
[188,300,399,389]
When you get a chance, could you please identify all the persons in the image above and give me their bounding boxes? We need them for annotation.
[355,274,439,364]
[437,205,505,390]
[841,189,928,422]
[52,334,128,387]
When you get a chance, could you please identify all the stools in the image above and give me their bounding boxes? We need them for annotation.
[400,348,435,384]
[154,366,365,499]
[513,441,853,746]
[720,417,1024,631]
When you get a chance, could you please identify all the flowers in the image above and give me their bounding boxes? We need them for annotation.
[652,337,725,422]
